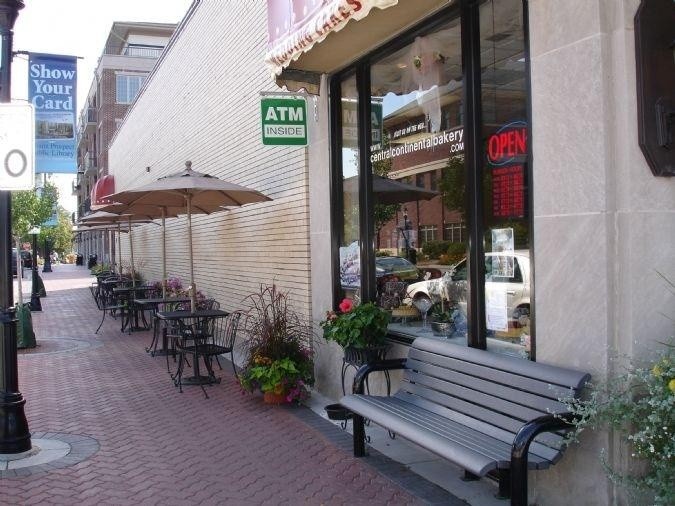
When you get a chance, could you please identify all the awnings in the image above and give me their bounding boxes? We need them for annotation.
[89,176,115,210]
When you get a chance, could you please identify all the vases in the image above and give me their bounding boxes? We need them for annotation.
[263,390,295,404]
[338,343,393,365]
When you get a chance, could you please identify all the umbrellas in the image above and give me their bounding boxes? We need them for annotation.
[342,172,442,205]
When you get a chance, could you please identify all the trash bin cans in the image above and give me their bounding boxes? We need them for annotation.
[77,255,83,266]
[89,255,96,268]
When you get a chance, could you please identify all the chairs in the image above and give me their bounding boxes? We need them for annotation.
[89,270,245,400]
[339,336,592,506]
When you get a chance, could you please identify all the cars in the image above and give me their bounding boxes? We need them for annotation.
[403,249,532,329]
[12,247,23,278]
[22,251,32,269]
[340,256,425,288]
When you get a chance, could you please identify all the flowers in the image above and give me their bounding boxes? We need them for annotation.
[532,263,675,506]
[319,297,393,351]
[222,273,326,407]
